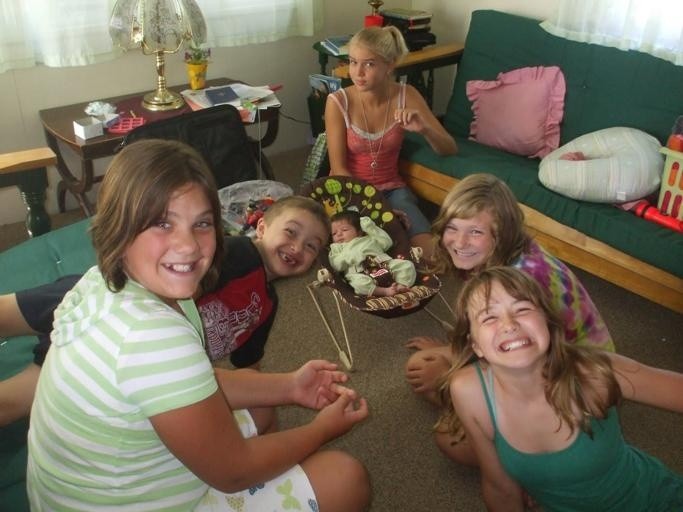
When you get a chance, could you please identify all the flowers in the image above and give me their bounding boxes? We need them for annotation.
[180,39,220,66]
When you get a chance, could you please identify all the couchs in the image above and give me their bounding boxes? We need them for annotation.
[310,8,683,323]
[0,211,109,512]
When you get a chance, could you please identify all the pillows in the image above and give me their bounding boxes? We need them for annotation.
[533,121,666,206]
[452,60,571,163]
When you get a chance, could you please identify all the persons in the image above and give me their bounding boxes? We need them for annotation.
[0,194,332,436]
[327,210,417,298]
[27,139,372,512]
[402,173,616,467]
[425,266,682,511]
[324,25,458,263]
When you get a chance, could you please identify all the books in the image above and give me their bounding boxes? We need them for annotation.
[320,32,352,56]
[202,87,241,106]
[380,6,434,32]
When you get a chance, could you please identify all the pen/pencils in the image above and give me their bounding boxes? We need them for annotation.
[129,110,136,118]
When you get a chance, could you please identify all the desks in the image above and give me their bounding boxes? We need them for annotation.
[311,31,466,129]
[37,74,280,220]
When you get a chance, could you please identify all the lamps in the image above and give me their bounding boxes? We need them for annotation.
[104,1,221,117]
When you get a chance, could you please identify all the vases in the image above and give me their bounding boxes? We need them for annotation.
[184,62,213,91]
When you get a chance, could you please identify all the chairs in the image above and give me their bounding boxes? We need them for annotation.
[109,101,276,192]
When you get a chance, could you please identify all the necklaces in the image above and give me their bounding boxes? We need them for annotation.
[358,81,392,170]
